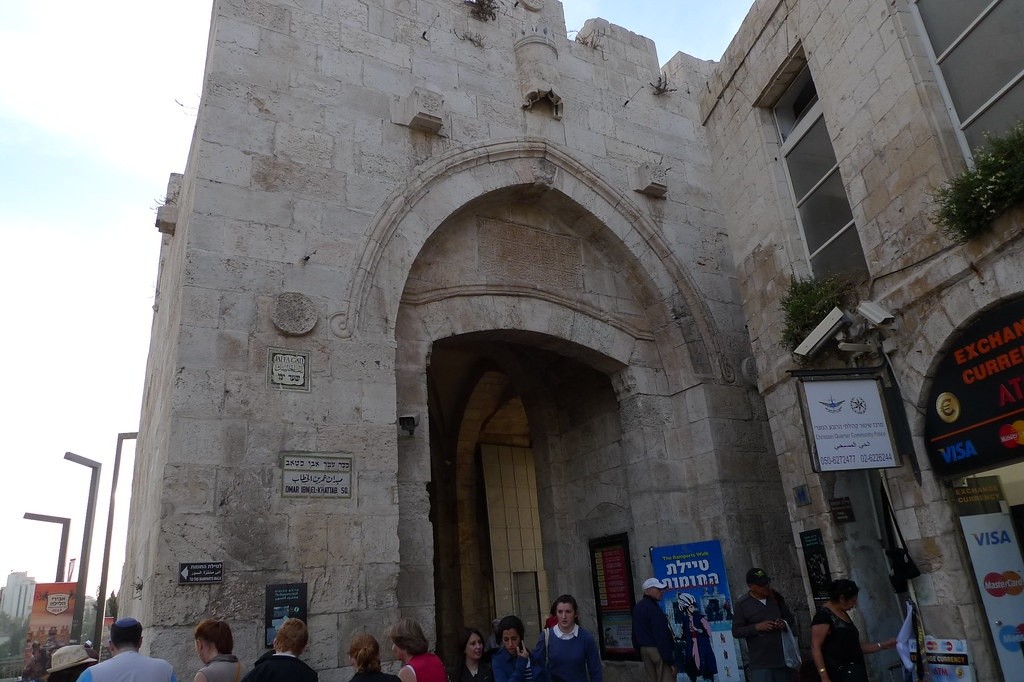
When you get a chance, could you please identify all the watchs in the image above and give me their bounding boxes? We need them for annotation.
[818,667,826,672]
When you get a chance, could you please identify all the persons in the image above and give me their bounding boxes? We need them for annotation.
[631,578,677,682]
[348,634,402,682]
[449,628,495,682]
[194,619,240,682]
[241,618,318,682]
[487,619,502,650]
[389,617,447,682]
[732,567,795,682]
[811,579,898,682]
[77,617,177,682]
[492,615,545,682]
[20,639,99,682]
[532,595,603,682]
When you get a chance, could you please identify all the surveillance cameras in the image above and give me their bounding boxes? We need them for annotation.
[399,413,421,431]
[794,306,856,362]
[856,301,895,328]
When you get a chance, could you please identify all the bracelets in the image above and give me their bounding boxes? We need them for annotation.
[877,642,882,650]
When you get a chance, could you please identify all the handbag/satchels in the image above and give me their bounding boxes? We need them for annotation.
[781,620,802,671]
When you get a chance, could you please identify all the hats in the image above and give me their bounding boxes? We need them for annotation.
[47,644,98,674]
[642,578,667,589]
[746,568,770,584]
